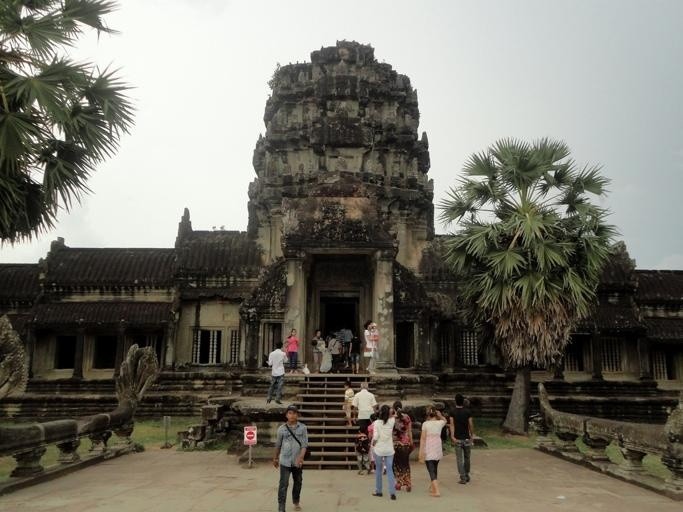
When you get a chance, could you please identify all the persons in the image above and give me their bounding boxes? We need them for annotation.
[264,341,289,405]
[272,403,308,511]
[309,318,379,374]
[283,327,303,375]
[342,380,448,500]
[448,393,475,485]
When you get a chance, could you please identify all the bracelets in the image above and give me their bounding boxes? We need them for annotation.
[273,458,279,461]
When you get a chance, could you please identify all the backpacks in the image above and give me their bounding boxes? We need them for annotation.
[356,436,369,453]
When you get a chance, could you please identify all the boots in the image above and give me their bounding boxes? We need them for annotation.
[429,480,439,497]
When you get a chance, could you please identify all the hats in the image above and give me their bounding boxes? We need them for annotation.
[287,405,298,413]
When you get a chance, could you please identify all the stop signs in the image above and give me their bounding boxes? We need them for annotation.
[245,431,254,439]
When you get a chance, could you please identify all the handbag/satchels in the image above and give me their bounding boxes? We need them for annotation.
[364,352,373,358]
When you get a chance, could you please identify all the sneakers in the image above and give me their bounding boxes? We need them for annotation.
[366,368,375,375]
[294,502,301,511]
[456,480,466,484]
[390,494,396,499]
[266,400,271,405]
[373,492,382,496]
[275,400,282,405]
